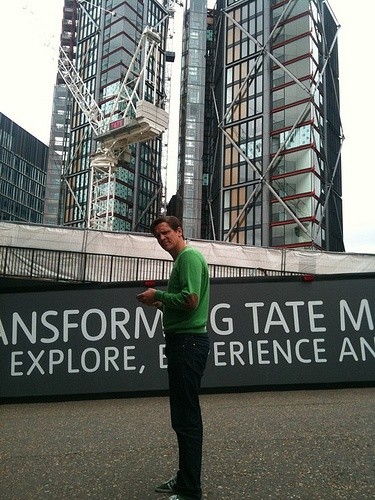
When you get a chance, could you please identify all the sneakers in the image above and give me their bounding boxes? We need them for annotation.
[167,492,192,500]
[156,475,181,493]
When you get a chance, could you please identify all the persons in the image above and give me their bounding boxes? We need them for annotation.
[135,216,210,500]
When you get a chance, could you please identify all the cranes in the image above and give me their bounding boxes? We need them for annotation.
[22,2,175,231]
